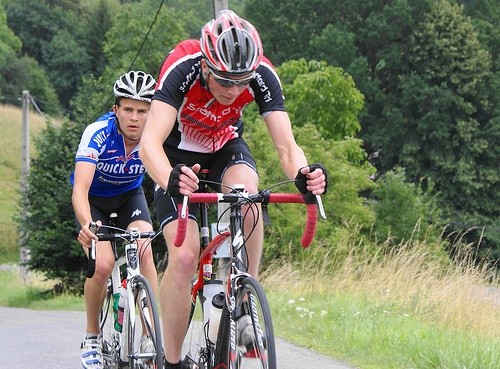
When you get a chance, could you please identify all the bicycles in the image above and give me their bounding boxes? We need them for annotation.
[86,220,163,369]
[173,163,328,369]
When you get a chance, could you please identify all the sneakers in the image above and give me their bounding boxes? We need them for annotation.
[81,336,104,369]
[138,336,156,362]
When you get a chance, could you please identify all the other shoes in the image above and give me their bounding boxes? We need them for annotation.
[237,315,266,351]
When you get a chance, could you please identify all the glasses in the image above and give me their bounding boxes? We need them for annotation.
[207,66,256,87]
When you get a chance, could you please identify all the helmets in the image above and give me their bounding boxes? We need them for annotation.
[113,71,157,103]
[199,9,264,75]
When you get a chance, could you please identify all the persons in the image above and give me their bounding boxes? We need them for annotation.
[70,71,164,369]
[139,9,329,369]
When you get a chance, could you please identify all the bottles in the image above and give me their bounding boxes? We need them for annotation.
[112,285,122,331]
[202,274,224,339]
[209,292,226,347]
[117,279,128,326]
